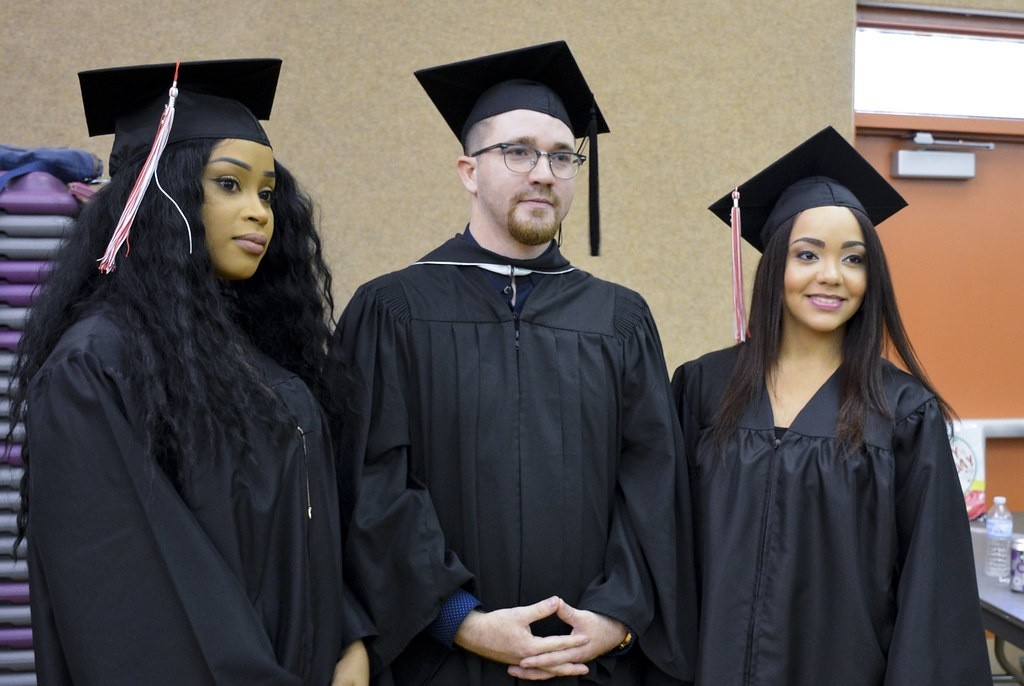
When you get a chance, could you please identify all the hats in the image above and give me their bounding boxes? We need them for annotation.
[707,125,909,345]
[413,39,611,258]
[78,58,283,274]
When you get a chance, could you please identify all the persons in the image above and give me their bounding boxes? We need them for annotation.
[671,125,995,686]
[0,58,372,686]
[322,40,678,686]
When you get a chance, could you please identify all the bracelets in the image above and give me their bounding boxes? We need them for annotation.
[619,634,632,649]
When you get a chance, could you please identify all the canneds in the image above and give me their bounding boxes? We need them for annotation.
[1009,538,1024,593]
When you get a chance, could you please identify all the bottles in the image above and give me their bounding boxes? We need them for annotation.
[984,496,1014,578]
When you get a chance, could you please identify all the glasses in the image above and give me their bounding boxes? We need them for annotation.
[471,143,586,181]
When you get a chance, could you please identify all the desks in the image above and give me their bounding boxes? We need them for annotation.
[969,509,1024,686]
